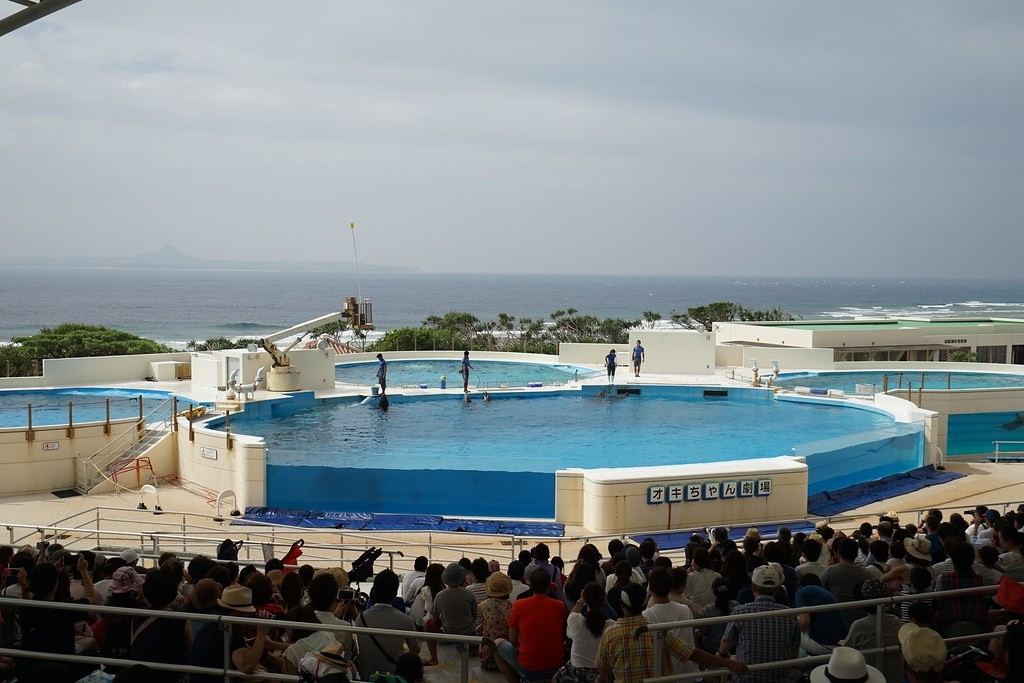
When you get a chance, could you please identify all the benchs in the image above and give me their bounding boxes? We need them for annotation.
[987,440,1024,463]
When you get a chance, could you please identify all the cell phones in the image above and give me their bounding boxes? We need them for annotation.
[336,589,356,600]
[61,555,79,566]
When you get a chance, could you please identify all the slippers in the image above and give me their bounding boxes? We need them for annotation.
[421,660,434,666]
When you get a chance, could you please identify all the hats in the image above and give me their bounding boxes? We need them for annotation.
[120,549,139,563]
[816,521,827,529]
[827,532,846,551]
[524,564,539,584]
[17,544,40,559]
[743,527,762,540]
[983,509,1000,521]
[326,567,349,587]
[217,585,256,612]
[441,563,467,586]
[489,560,500,572]
[485,571,513,597]
[767,562,785,584]
[751,565,780,588]
[267,569,284,586]
[621,582,648,607]
[885,511,899,525]
[315,645,348,673]
[810,647,886,683]
[898,623,948,673]
[109,565,145,594]
[903,535,932,561]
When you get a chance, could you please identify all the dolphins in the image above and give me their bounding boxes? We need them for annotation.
[463,391,471,403]
[483,390,492,401]
[379,392,389,408]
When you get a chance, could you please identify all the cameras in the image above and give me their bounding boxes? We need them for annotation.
[8,568,21,576]
[964,510,975,516]
[980,518,986,523]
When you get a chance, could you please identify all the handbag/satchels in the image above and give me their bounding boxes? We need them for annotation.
[395,653,424,683]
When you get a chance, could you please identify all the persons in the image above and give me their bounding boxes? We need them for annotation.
[632,340,644,377]
[605,349,617,385]
[1,505,1024,683]
[462,351,474,392]
[376,353,387,395]
